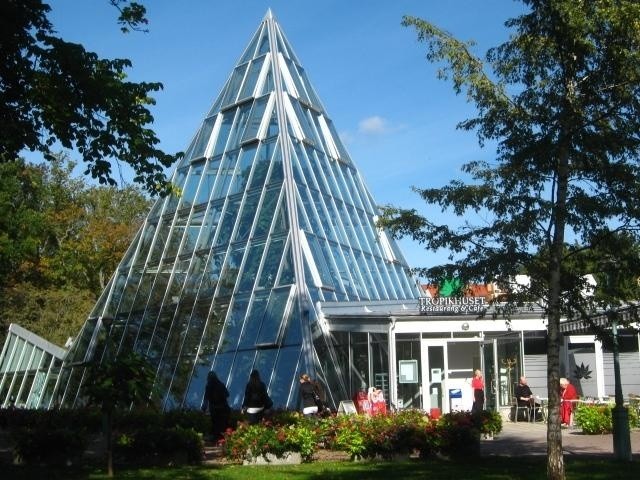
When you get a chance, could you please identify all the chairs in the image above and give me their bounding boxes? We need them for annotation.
[511,395,582,427]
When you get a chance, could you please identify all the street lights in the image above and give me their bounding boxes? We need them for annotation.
[102,316,112,363]
[600,255,631,461]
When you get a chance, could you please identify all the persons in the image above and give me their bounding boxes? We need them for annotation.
[197,370,232,441]
[514,376,539,421]
[299,372,321,417]
[559,377,577,428]
[239,369,273,427]
[471,368,487,412]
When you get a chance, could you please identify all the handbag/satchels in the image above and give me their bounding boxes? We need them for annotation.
[312,393,323,408]
